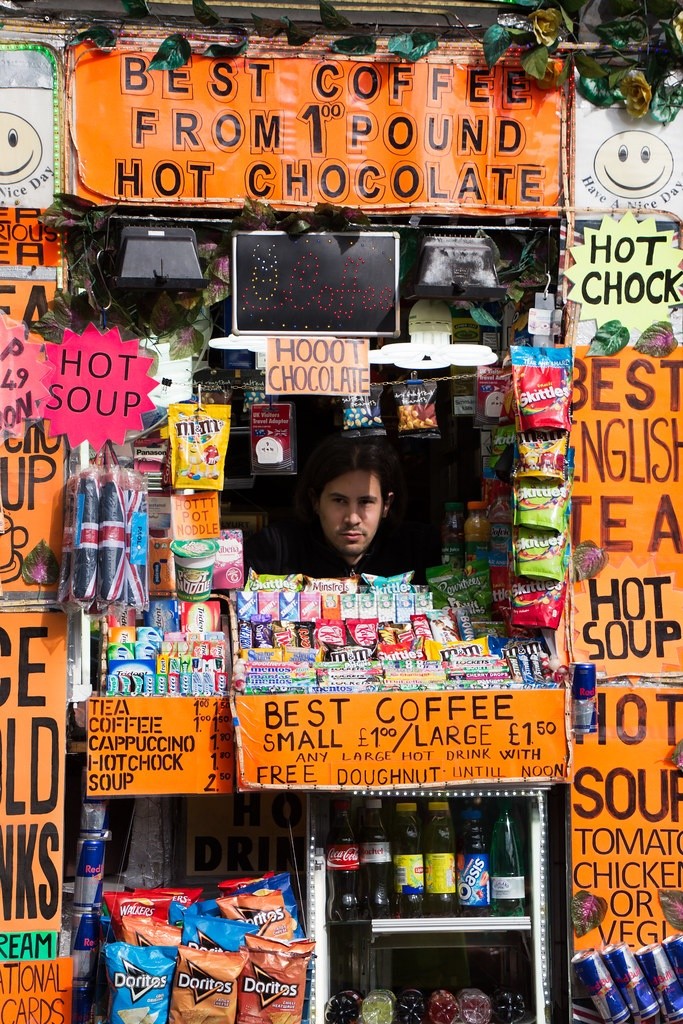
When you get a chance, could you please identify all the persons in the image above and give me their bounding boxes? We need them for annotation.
[244,437,438,585]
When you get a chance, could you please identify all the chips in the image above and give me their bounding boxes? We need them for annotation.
[117,1007,298,1024]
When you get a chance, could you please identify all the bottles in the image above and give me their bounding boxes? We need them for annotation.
[440,495,512,567]
[326,800,526,922]
[324,988,523,1024]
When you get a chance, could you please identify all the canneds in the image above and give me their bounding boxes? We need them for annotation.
[70,763,106,1024]
[570,933,683,1024]
[568,662,598,735]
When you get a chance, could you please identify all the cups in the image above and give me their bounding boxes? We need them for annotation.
[170,538,219,602]
